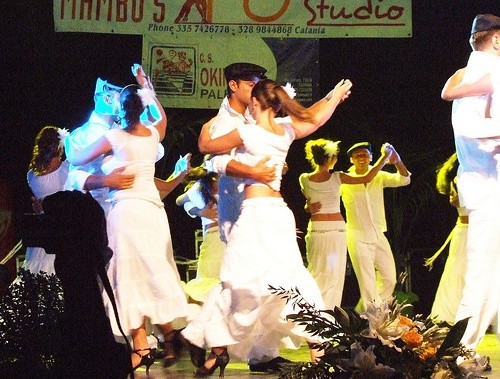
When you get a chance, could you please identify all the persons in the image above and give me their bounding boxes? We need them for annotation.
[424,152,469,324]
[8,126,69,316]
[64,62,221,374]
[452,13,500,371]
[193,79,352,377]
[441,63,500,123]
[449,191,460,207]
[304,141,412,315]
[298,138,393,365]
[178,62,351,371]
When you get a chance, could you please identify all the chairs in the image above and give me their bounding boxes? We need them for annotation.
[185,229,202,283]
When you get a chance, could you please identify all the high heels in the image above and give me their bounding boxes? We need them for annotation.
[132,348,156,374]
[194,348,230,378]
[164,329,187,365]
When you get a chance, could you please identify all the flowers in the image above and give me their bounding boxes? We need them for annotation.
[268,272,492,379]
[322,141,341,158]
[57,128,70,150]
[281,81,297,100]
[136,87,158,108]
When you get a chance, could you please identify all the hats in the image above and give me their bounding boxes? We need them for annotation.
[347,142,371,156]
[225,63,268,82]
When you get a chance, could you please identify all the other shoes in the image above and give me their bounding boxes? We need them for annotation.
[192,343,206,369]
[249,356,298,372]
